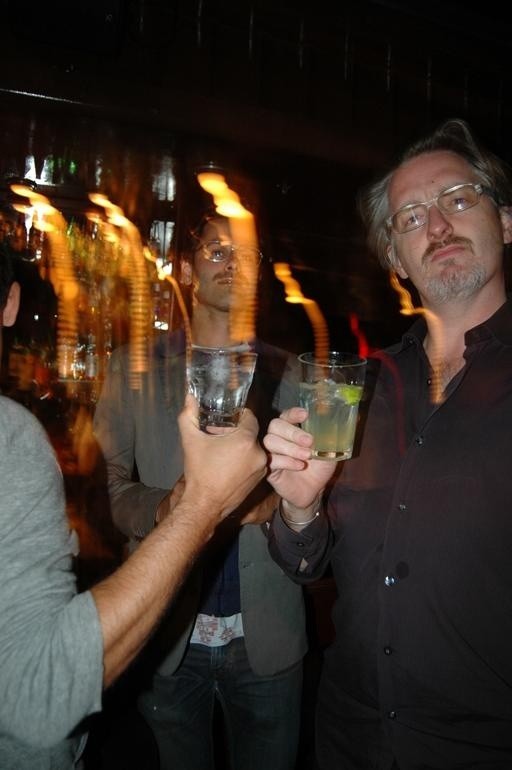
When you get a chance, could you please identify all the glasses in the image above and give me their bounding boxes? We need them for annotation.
[381,180,507,243]
[190,236,264,270]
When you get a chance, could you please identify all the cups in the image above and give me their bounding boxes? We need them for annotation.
[188,347,260,436]
[295,350,368,462]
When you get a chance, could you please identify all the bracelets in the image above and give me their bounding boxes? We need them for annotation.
[279,503,320,526]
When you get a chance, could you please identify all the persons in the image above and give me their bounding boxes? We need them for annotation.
[1,223,272,767]
[90,195,313,769]
[261,114,510,767]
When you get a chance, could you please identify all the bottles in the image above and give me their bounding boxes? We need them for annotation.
[0,104,143,474]
